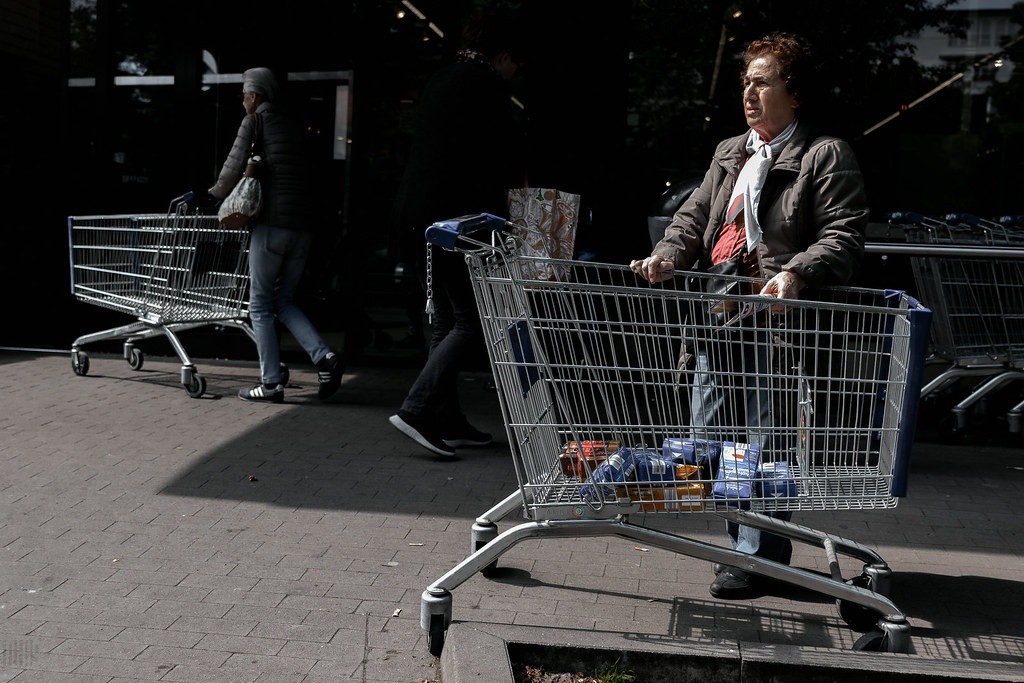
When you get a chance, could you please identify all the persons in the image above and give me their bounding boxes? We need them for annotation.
[190,67,344,406]
[630,36,868,599]
[386,35,531,455]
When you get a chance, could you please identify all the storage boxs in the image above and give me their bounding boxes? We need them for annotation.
[559,436,797,511]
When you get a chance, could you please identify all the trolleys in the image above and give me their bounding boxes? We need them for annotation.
[846,211,1024,443]
[67,192,289,399]
[419,215,934,659]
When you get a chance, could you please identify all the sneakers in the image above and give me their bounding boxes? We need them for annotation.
[239,382,284,404]
[317,350,345,402]
[708,560,781,599]
[389,410,457,456]
[441,421,493,447]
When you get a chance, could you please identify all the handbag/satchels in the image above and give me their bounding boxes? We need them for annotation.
[217,112,264,229]
[506,184,582,285]
[695,247,752,315]
